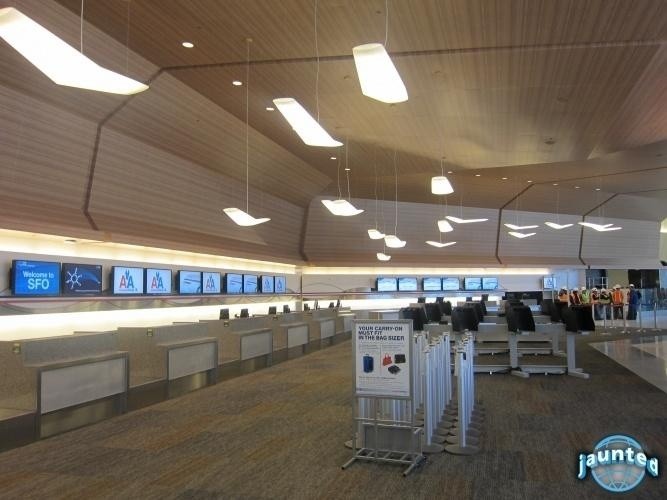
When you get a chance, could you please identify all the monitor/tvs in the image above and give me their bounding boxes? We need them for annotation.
[223,273,242,295]
[175,270,202,296]
[201,272,221,294]
[273,276,286,293]
[62,263,102,296]
[242,274,258,294]
[378,277,498,292]
[12,260,61,297]
[144,268,172,294]
[111,266,143,296]
[258,275,274,293]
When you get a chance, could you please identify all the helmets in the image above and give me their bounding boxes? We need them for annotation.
[561,284,634,292]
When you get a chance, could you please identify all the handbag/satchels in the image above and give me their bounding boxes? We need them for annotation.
[383,352,392,366]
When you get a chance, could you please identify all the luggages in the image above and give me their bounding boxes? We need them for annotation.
[363,353,373,372]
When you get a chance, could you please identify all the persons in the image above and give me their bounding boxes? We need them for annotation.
[559,284,642,320]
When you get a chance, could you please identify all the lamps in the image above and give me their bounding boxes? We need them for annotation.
[321,126,365,218]
[222,37,271,226]
[504,176,623,240]
[0,0,148,95]
[272,0,344,148]
[423,156,488,248]
[353,1,408,103]
[368,139,406,262]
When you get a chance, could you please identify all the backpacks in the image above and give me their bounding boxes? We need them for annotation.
[638,292,641,299]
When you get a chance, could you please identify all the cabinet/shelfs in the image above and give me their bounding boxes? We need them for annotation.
[424,310,589,379]
